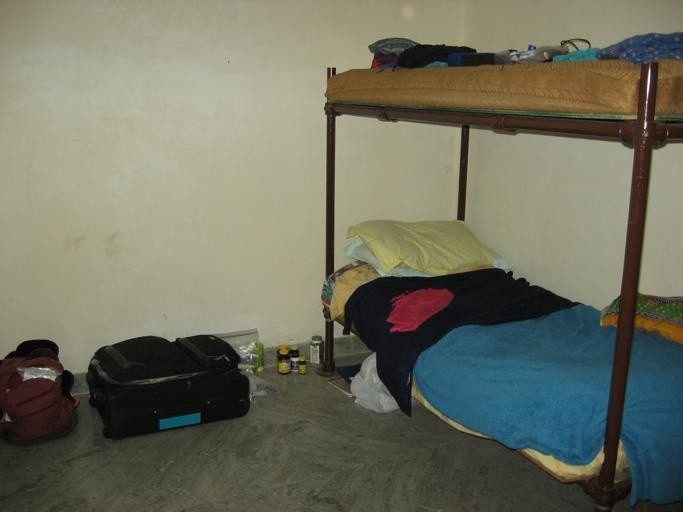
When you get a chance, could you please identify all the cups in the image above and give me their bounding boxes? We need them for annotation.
[255,342,265,374]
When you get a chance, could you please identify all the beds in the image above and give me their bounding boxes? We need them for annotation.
[323,59,683,512]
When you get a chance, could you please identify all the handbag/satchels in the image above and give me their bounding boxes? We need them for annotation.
[0,339,78,445]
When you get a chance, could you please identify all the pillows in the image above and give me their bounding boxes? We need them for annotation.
[346,235,513,277]
[349,221,499,277]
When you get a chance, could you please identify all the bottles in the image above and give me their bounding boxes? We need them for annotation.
[276,336,325,375]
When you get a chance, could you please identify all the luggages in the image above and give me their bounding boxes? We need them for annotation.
[86,334,250,441]
